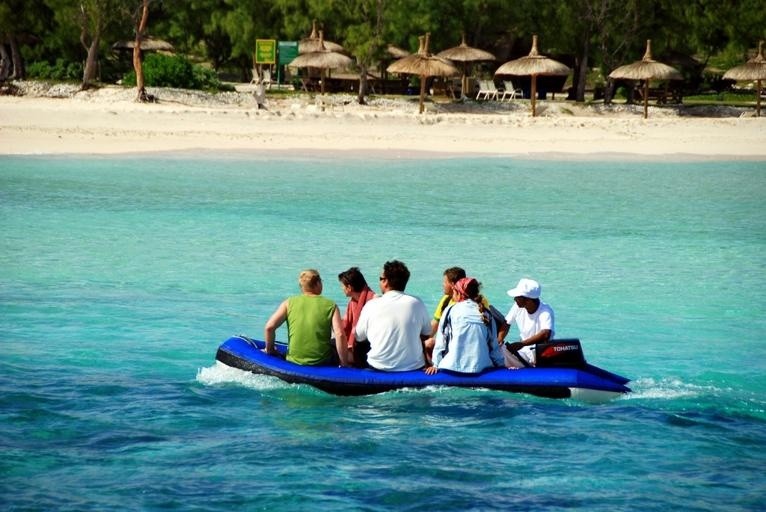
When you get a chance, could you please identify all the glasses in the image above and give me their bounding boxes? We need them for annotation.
[379,277,387,280]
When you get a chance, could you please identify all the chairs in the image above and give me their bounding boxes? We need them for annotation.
[250,69,262,85]
[475,79,524,102]
[259,69,279,86]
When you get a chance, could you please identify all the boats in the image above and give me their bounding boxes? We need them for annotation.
[215,336,632,406]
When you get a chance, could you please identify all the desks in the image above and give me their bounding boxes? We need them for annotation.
[298,78,310,92]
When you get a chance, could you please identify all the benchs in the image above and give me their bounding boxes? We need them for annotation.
[642,89,665,99]
[375,79,409,94]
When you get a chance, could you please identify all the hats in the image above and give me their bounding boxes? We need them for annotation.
[506,278,541,299]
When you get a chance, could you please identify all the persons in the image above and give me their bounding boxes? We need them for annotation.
[496,278,556,369]
[259,269,350,368]
[423,265,511,366]
[351,259,434,373]
[421,277,520,376]
[338,267,374,368]
